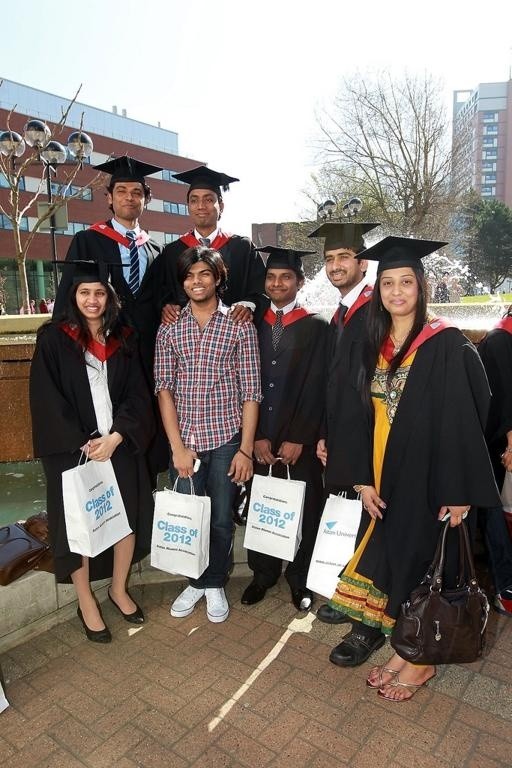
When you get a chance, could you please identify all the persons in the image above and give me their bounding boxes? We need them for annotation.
[242,247,329,609]
[156,244,261,621]
[16,298,54,316]
[354,236,489,701]
[54,155,161,304]
[139,166,262,348]
[422,266,464,303]
[33,258,145,644]
[314,222,385,666]
[477,305,511,615]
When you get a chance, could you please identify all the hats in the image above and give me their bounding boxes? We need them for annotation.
[52,258,132,286]
[253,245,317,273]
[354,235,449,277]
[92,155,163,186]
[307,223,381,252]
[171,165,239,195]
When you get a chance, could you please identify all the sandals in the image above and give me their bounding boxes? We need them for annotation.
[378,665,436,702]
[367,653,399,689]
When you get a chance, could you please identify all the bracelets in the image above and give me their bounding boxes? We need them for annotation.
[350,482,367,491]
[239,448,254,465]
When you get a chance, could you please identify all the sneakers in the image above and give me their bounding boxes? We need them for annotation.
[205,587,230,624]
[171,584,205,618]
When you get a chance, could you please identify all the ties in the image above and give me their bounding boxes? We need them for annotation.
[272,310,284,351]
[126,232,140,299]
[199,238,209,248]
[337,303,348,341]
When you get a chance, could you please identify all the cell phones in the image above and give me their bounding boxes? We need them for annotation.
[193,458,201,473]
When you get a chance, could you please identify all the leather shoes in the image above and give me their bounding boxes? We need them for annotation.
[77,603,111,643]
[241,578,269,604]
[330,630,386,668]
[284,569,313,611]
[107,587,144,623]
[317,604,355,623]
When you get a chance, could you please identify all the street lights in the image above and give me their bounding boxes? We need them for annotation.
[318,198,363,223]
[0,120,93,297]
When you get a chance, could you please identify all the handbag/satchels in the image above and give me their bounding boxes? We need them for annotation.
[305,493,362,600]
[151,487,211,580]
[62,459,133,558]
[1,523,51,586]
[391,586,491,664]
[243,475,306,562]
